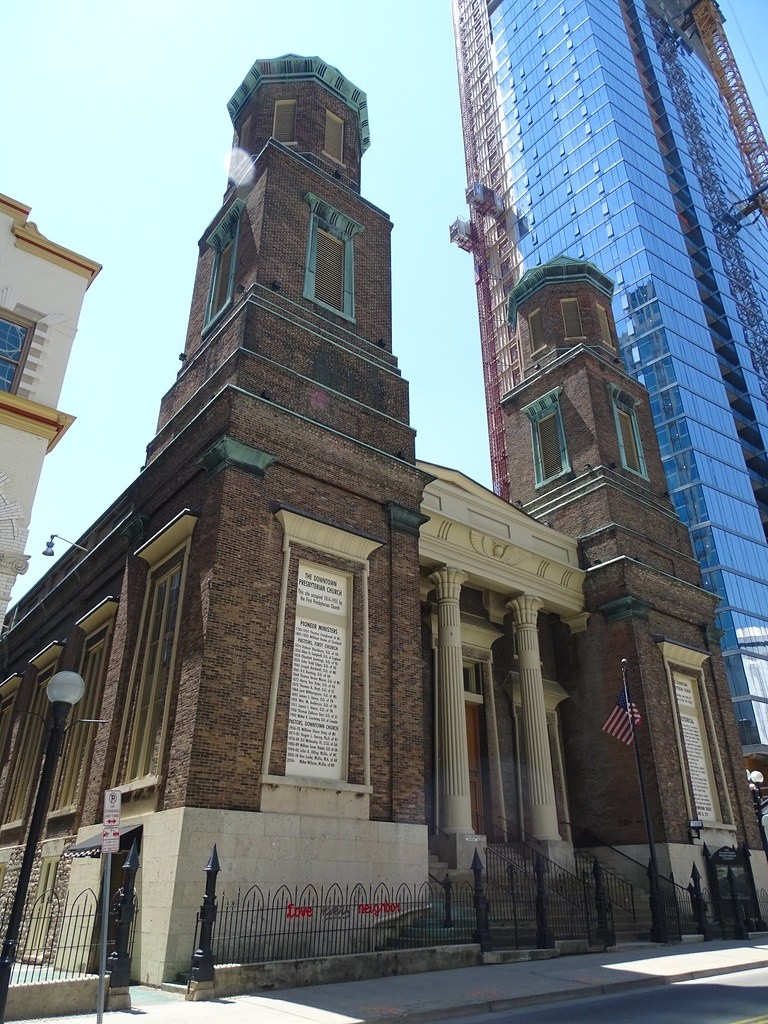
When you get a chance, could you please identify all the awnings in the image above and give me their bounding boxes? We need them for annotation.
[64,823,143,857]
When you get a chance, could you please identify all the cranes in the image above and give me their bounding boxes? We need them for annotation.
[648,10,768,410]
[689,0,768,226]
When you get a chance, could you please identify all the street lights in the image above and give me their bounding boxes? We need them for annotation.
[0,670,85,1024]
[748,770,768,863]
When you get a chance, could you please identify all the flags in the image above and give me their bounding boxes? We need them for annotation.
[601,687,641,749]
